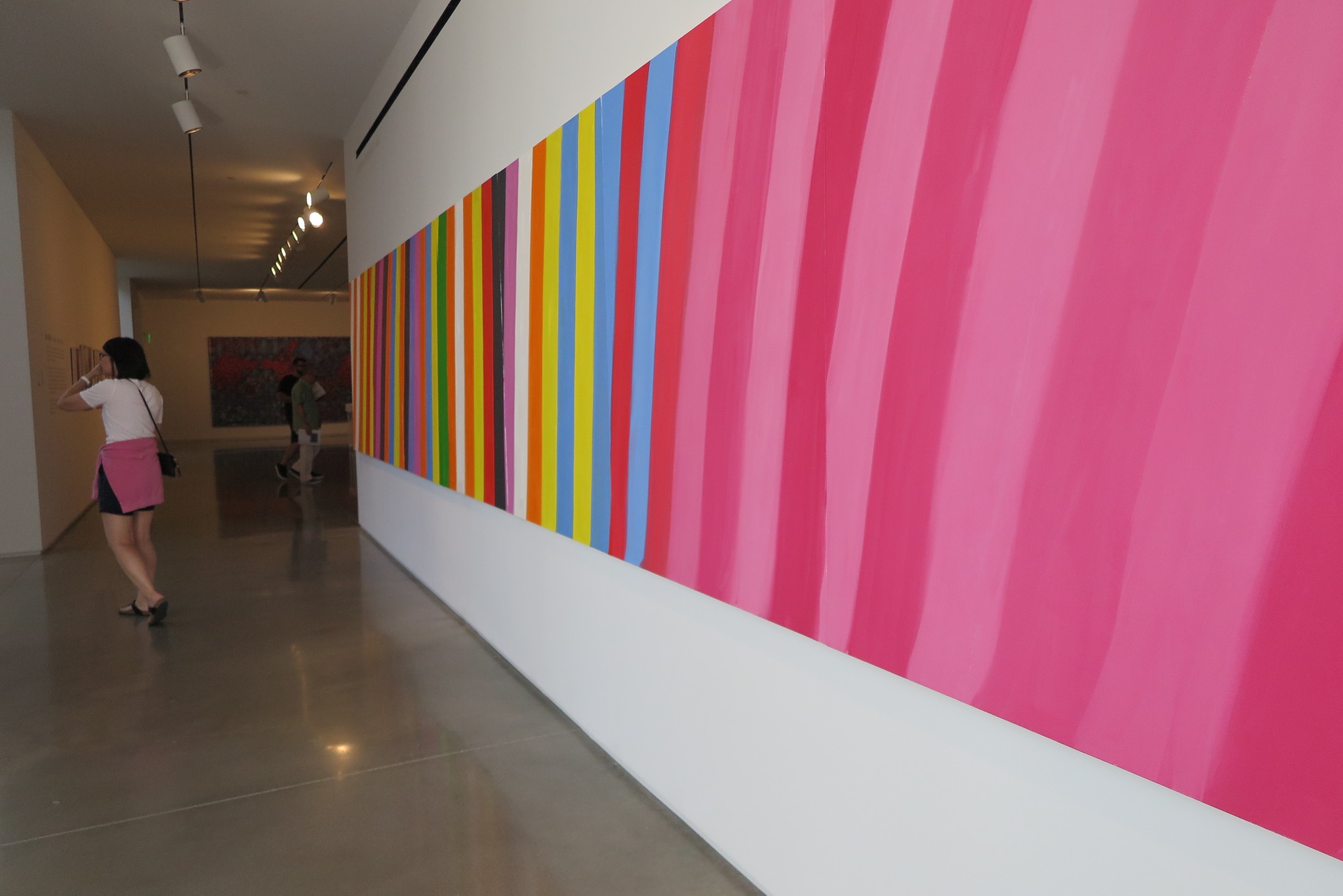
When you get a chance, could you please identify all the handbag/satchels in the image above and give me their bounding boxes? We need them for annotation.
[157,452,181,477]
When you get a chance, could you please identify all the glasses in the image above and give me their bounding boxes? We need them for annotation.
[99,353,110,361]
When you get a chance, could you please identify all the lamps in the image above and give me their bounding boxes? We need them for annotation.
[196,290,206,303]
[170,90,203,134]
[257,290,269,303]
[163,23,202,77]
[306,185,330,209]
[271,206,323,275]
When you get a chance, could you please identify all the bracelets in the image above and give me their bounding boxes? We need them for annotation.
[80,376,90,386]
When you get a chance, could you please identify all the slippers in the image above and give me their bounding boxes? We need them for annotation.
[147,597,168,627]
[118,599,151,617]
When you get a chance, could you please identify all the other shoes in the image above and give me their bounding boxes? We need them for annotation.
[275,463,288,480]
[310,471,324,479]
[306,478,322,484]
[289,467,301,480]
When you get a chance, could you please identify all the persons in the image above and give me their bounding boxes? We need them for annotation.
[57,337,169,626]
[275,357,308,479]
[292,368,324,485]
